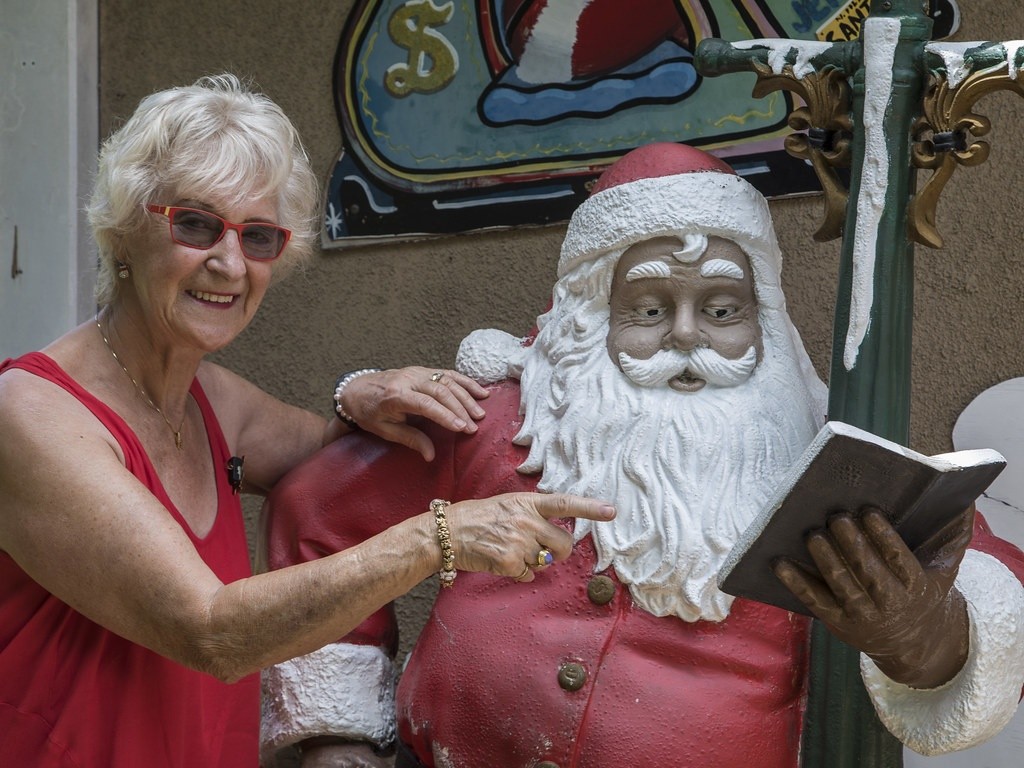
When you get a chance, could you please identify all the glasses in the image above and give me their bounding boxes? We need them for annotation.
[143,200,292,265]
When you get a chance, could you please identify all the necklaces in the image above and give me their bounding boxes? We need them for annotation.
[96,312,187,449]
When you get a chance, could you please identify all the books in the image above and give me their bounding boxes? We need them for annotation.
[717,421,1008,620]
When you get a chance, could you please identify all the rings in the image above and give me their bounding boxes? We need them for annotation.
[532,548,552,570]
[514,566,528,580]
[431,372,444,382]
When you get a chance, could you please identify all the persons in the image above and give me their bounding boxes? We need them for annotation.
[0,71,616,768]
[260,145,1024,768]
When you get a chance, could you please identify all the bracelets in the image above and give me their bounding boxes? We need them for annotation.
[333,367,385,429]
[429,498,457,588]
